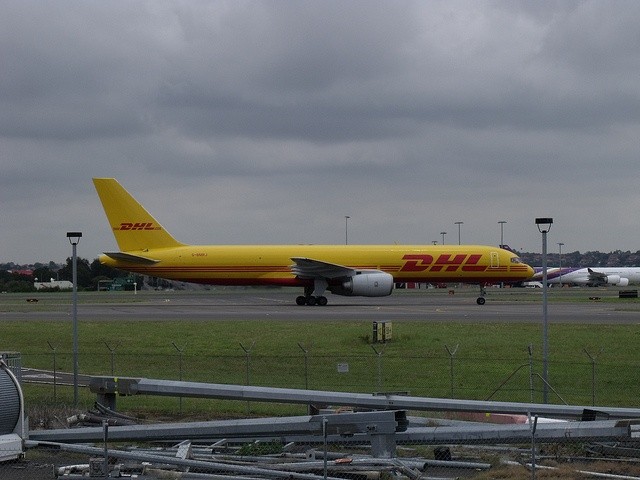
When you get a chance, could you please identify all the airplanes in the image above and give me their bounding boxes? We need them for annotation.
[524,266,640,288]
[90,178,536,305]
